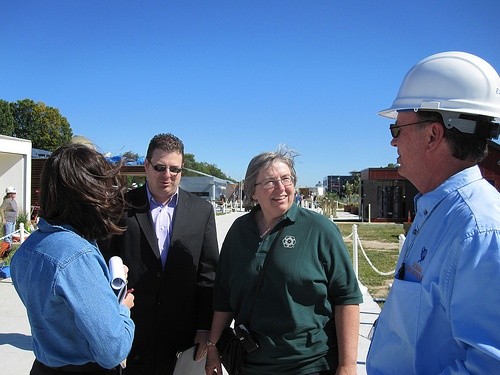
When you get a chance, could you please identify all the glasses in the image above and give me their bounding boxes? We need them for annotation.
[251,175,295,189]
[390,120,443,138]
[146,159,184,173]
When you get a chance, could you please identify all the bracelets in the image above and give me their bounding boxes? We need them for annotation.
[207,340,215,346]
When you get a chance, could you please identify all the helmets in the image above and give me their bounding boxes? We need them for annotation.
[5,187,17,193]
[376,51,500,119]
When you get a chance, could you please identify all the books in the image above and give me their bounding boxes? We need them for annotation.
[109,256,129,368]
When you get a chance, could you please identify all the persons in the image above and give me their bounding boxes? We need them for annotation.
[205,152,363,375]
[96,133,219,375]
[366,51,500,375]
[0,186,18,244]
[10,144,135,375]
